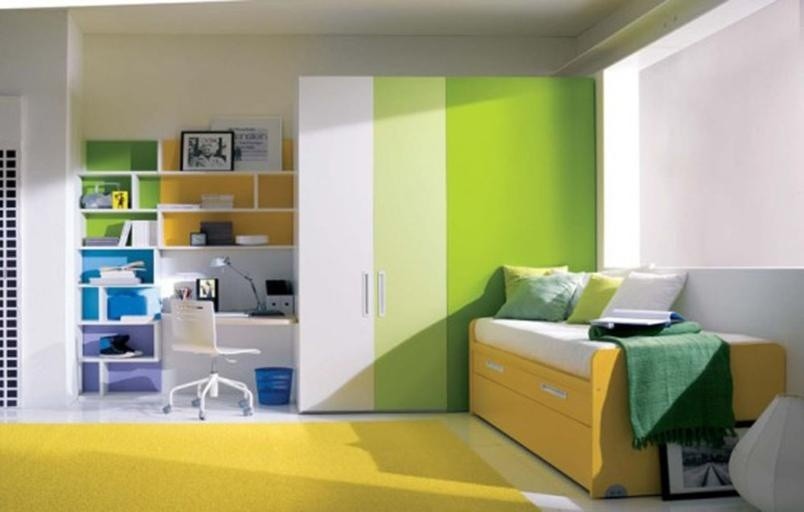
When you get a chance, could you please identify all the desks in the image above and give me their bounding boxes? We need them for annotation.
[160,310,299,327]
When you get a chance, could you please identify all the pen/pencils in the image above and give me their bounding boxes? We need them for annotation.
[178,288,192,299]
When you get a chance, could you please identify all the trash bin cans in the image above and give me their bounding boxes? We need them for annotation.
[255,367,292,405]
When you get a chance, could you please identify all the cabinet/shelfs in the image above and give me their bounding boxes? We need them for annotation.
[77,139,162,406]
[471,344,593,498]
[159,138,295,248]
[292,73,598,414]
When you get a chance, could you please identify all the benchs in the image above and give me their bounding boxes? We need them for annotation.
[468,315,784,500]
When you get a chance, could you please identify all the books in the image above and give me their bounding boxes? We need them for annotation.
[588,308,686,327]
[80,193,270,324]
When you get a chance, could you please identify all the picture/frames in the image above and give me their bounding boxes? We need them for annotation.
[656,419,756,500]
[179,129,235,172]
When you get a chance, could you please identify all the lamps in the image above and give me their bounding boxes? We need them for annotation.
[211,256,268,313]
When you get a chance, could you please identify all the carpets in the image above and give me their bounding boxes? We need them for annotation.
[0,415,543,512]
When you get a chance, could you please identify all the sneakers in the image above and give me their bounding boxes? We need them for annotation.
[99,336,135,357]
[116,334,143,356]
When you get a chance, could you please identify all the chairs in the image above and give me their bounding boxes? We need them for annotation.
[163,299,261,416]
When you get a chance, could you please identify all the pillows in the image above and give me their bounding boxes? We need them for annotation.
[492,260,688,325]
